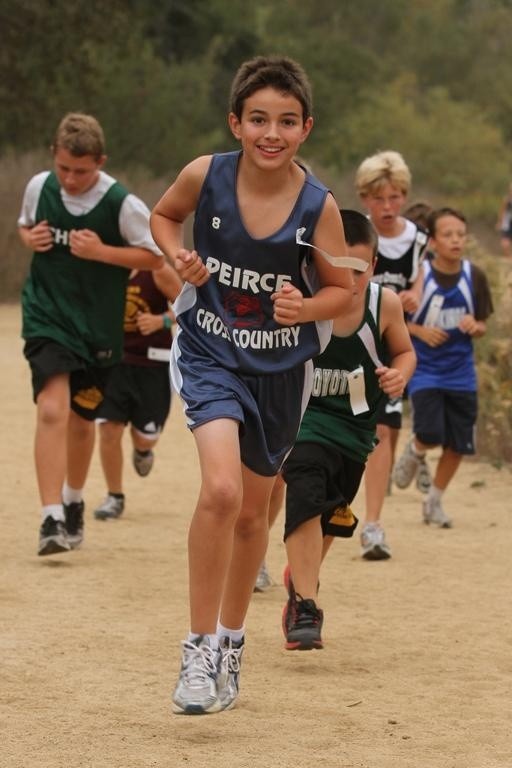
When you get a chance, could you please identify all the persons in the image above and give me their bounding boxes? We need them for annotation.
[353,149,426,558]
[94,263,185,519]
[253,473,286,592]
[387,202,432,494]
[16,112,164,556]
[394,207,495,528]
[150,52,354,715]
[281,208,418,649]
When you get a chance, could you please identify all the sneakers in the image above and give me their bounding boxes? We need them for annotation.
[133,449,154,476]
[360,522,391,560]
[37,516,71,556]
[285,561,320,602]
[218,636,246,711]
[392,439,454,528]
[254,559,272,592]
[92,496,124,520]
[170,634,222,715]
[62,500,85,548]
[284,599,324,652]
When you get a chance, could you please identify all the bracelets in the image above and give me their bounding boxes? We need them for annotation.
[161,312,171,330]
